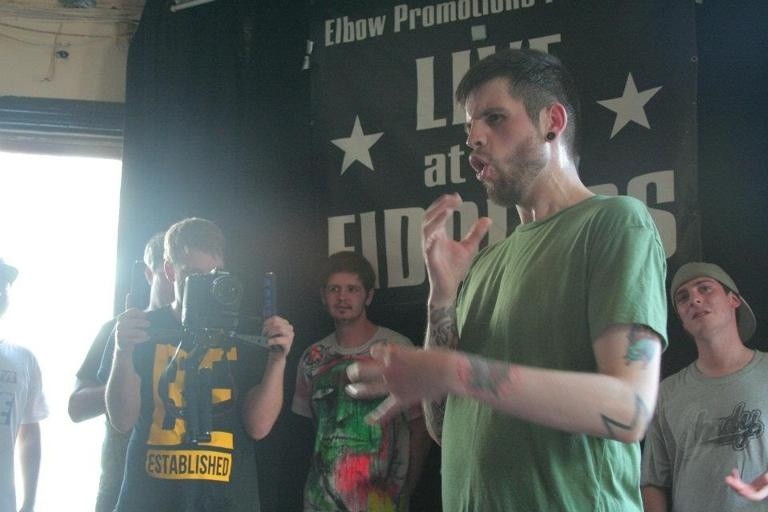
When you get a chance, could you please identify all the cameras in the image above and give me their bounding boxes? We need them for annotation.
[181,271,241,332]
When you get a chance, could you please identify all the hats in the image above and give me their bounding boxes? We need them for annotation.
[670,261,757,343]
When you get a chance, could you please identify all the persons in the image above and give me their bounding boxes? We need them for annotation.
[640,262,768,512]
[0,259,49,512]
[346,50,669,512]
